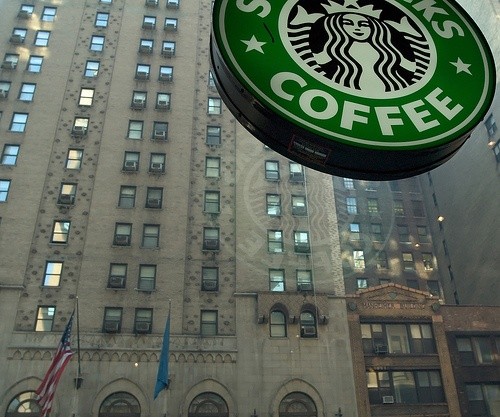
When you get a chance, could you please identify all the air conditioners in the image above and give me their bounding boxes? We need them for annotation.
[139,45,174,55]
[143,0,177,31]
[123,161,163,172]
[148,199,160,208]
[60,195,73,204]
[109,276,123,287]
[376,344,388,353]
[136,71,172,81]
[73,126,84,135]
[105,322,149,333]
[293,172,315,335]
[0,10,29,97]
[115,235,128,245]
[383,395,394,403]
[133,98,169,109]
[153,129,165,140]
[205,239,218,290]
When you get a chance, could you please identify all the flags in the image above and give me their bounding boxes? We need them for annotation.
[35,308,74,417]
[154,318,170,399]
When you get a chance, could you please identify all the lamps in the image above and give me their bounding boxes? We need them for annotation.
[487,132,496,146]
